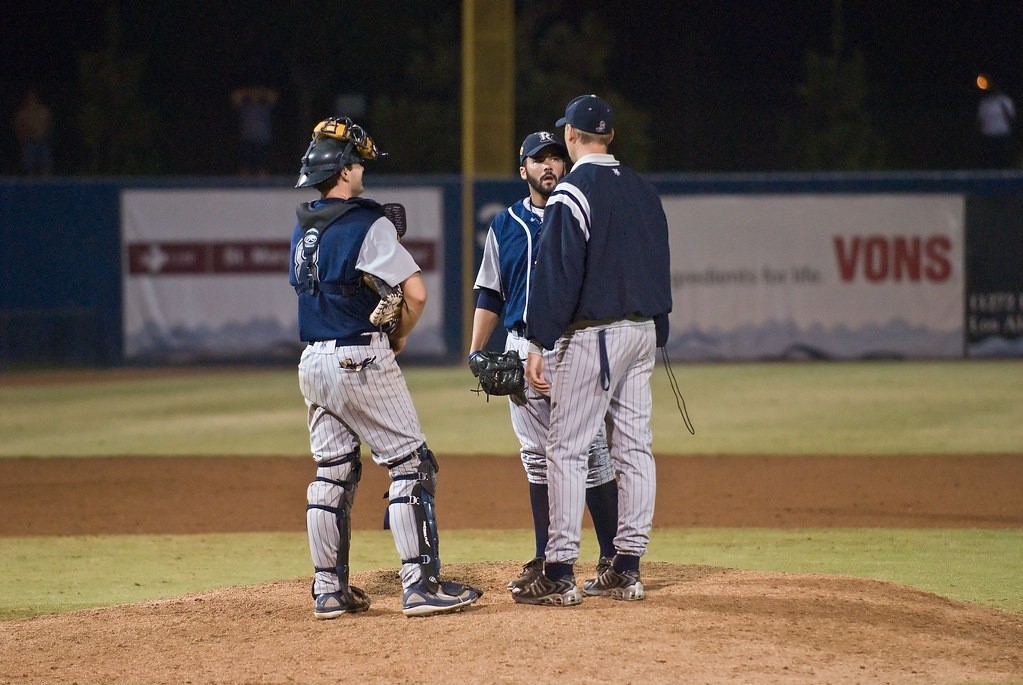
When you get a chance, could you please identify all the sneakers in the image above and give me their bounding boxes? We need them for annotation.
[596,557,612,577]
[582,555,645,602]
[511,570,582,607]
[506,558,544,590]
[403,580,479,618]
[315,587,371,620]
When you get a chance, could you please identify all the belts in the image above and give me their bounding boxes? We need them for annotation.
[308,335,372,347]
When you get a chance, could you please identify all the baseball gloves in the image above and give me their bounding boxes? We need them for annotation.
[466,349,528,403]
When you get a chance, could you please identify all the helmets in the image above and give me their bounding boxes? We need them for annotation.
[292,135,365,185]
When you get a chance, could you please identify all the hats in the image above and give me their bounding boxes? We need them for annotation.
[520,131,565,164]
[554,94,615,135]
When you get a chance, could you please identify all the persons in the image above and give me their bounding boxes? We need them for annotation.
[468,129,618,593]
[513,93,673,608]
[289,137,484,619]
